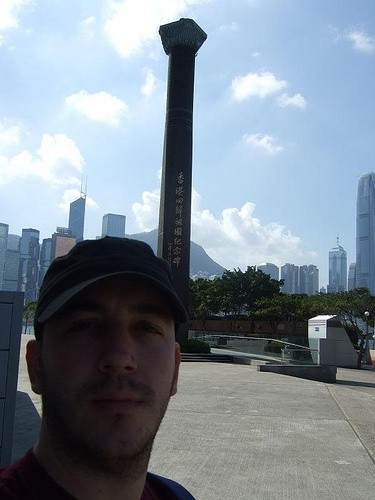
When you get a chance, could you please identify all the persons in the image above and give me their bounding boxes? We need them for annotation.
[0,237,196,500]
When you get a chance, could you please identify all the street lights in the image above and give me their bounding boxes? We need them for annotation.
[372,334,374,339]
[362,310,370,364]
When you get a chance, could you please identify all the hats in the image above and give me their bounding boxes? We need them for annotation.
[34,236,189,340]
